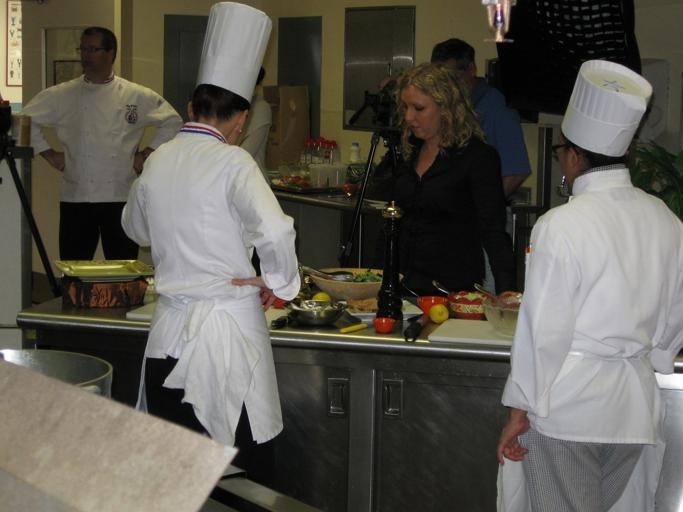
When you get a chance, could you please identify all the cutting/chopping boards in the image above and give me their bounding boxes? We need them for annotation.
[126,302,291,329]
[427,307,519,346]
[272,186,345,195]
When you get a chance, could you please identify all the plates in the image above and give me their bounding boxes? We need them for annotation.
[345,300,424,322]
[54,260,155,282]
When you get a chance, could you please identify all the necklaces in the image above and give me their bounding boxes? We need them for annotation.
[84,70,115,84]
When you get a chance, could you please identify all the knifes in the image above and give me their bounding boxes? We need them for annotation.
[405,313,431,341]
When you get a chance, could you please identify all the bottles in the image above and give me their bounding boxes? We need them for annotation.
[300,137,339,168]
[376,268,403,324]
[350,143,360,162]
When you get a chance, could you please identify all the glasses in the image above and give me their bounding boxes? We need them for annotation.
[75,47,107,55]
[550,143,574,161]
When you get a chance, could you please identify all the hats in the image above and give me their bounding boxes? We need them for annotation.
[561,59,653,158]
[195,1,273,106]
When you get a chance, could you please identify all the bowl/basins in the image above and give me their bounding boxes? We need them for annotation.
[328,165,342,188]
[310,268,404,301]
[417,296,449,316]
[447,291,493,320]
[308,164,328,189]
[283,300,348,326]
[483,300,519,339]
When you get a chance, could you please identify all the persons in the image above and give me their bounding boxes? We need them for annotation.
[497,129,683,512]
[226,68,273,166]
[120,85,302,478]
[20,26,183,260]
[431,39,531,198]
[376,62,507,297]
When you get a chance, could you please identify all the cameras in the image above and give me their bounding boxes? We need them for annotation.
[0,102,37,162]
[348,79,402,128]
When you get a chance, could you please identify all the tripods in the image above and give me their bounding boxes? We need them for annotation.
[339,133,417,270]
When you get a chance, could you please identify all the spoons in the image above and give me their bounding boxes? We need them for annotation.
[299,266,353,281]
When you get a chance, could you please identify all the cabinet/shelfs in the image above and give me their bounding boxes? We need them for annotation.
[18,282,681,511]
[269,188,356,268]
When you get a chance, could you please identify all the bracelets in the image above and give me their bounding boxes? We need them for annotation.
[140,151,148,160]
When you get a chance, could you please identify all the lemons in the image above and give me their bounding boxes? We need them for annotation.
[430,305,449,324]
